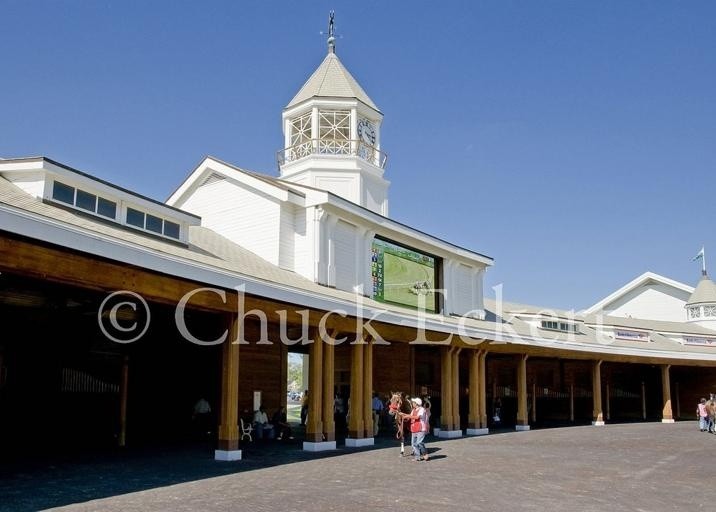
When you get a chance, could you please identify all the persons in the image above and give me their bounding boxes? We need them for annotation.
[696,398,709,432]
[252,390,431,443]
[404,397,430,461]
[192,396,212,420]
[492,396,502,425]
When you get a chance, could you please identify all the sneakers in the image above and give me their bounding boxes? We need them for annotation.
[424,454,428,460]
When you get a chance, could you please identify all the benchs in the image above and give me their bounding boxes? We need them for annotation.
[239,417,254,442]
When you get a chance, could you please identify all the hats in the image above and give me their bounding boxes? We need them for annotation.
[412,398,422,406]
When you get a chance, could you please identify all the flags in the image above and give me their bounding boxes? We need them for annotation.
[690,247,704,262]
